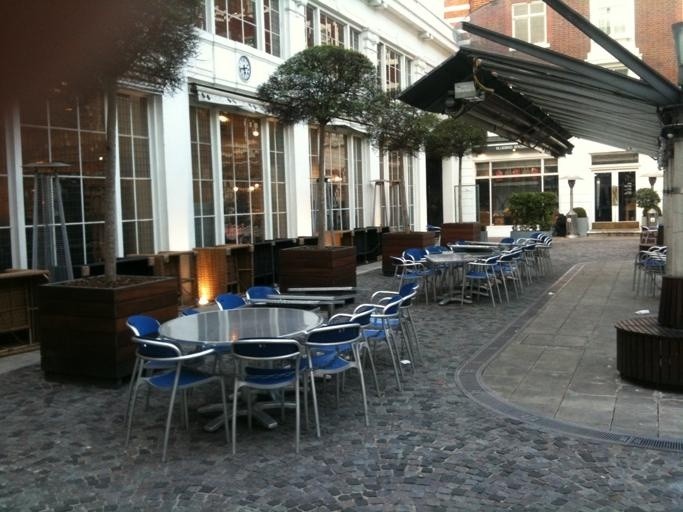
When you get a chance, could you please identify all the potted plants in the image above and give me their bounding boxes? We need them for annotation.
[572,208,588,234]
[506,192,557,242]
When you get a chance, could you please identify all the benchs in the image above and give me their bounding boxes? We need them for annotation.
[614,276,681,394]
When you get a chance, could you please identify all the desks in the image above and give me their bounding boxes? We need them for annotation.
[306,225,390,267]
[80,239,309,309]
[0,265,52,360]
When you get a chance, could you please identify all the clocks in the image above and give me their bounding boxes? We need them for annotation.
[236,54,251,83]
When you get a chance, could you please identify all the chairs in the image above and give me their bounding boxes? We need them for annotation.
[215,293,246,312]
[124,315,176,422]
[352,295,404,392]
[377,290,423,373]
[368,285,420,346]
[628,246,667,298]
[305,322,370,436]
[230,335,308,455]
[389,232,551,307]
[312,307,381,397]
[126,335,230,462]
[246,285,280,300]
